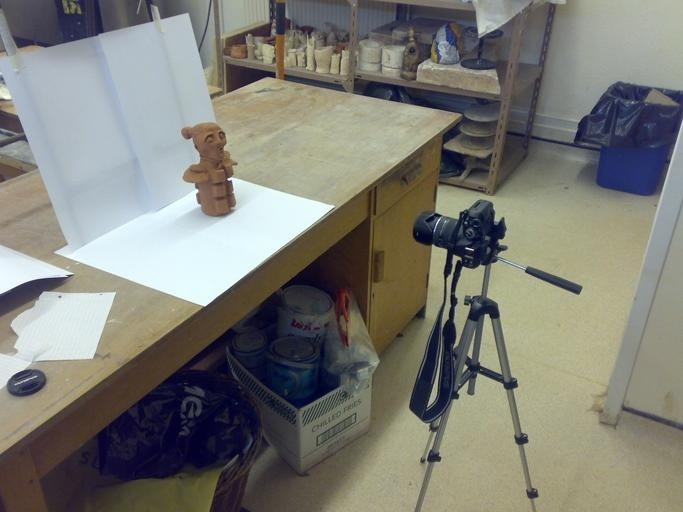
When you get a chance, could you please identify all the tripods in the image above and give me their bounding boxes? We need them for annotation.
[415,255,584,510]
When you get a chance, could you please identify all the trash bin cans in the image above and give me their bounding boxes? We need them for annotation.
[573,80,683,198]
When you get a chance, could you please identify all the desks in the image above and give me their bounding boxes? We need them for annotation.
[0,77,462,512]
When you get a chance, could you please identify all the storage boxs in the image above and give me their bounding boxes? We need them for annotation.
[226,300,374,475]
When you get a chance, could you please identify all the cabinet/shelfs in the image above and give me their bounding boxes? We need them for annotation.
[212,0,557,196]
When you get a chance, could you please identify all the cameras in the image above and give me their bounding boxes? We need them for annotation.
[413,198,509,270]
